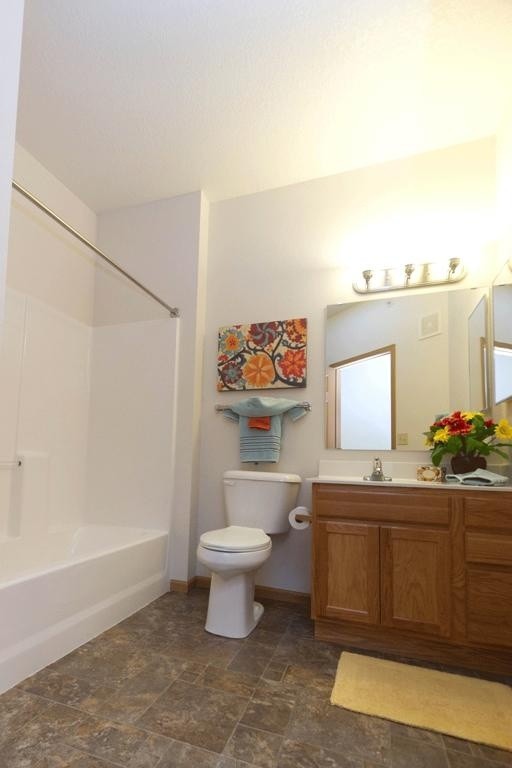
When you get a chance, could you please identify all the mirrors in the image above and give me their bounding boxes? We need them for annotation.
[491,253,512,408]
[321,281,489,450]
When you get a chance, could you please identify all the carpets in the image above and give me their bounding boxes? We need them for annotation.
[330,650,512,749]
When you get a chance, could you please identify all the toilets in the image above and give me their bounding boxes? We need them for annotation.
[195,469,301,638]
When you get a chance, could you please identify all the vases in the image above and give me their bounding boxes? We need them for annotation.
[448,454,488,477]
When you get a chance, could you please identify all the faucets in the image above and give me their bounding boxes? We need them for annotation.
[370,457,387,480]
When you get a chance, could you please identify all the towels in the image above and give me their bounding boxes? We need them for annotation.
[220,395,308,462]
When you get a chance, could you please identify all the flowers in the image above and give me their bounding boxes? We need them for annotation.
[423,403,512,467]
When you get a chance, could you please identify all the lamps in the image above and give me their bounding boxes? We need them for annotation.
[351,253,473,294]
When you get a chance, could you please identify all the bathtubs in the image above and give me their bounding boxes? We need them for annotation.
[0,523,167,697]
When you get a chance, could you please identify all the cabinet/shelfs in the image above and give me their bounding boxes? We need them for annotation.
[314,487,509,662]
